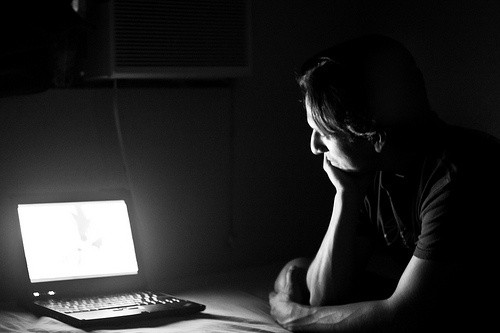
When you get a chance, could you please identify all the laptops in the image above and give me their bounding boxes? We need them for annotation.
[9,190,206,330]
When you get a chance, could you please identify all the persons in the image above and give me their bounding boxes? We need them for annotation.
[269,35,500,333]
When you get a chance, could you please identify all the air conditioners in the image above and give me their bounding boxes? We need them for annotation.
[80,0,254,80]
